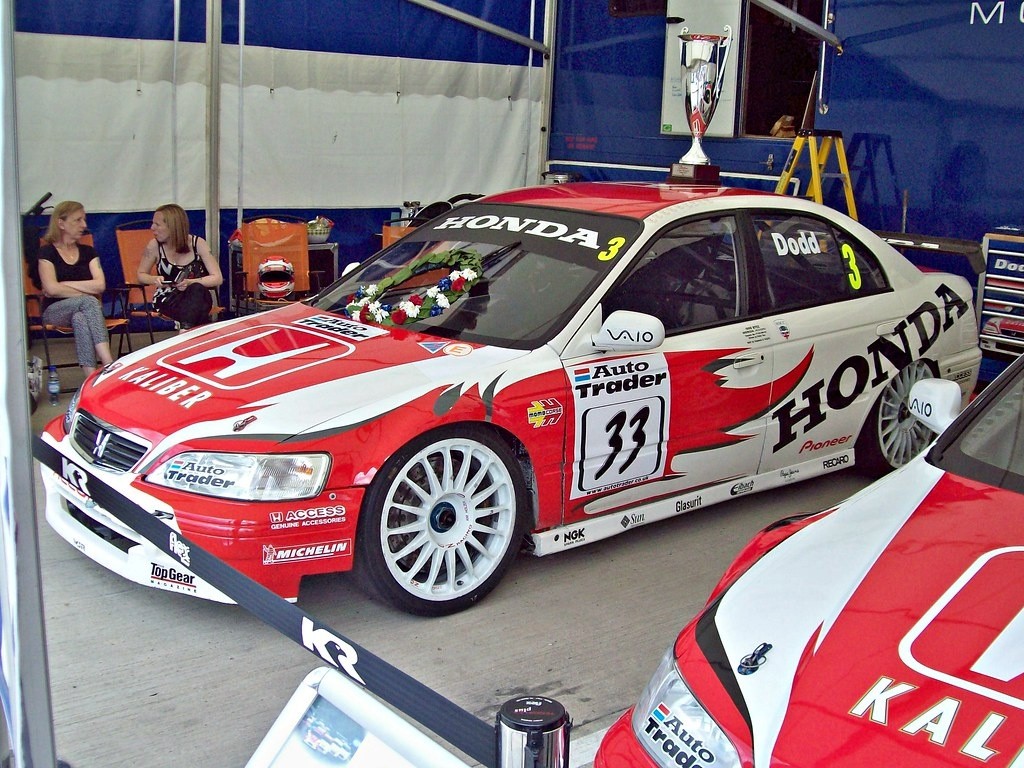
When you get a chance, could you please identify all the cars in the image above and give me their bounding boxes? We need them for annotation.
[38,183,982,616]
[594,352,1024,768]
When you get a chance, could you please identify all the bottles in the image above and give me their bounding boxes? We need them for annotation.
[46,366,61,407]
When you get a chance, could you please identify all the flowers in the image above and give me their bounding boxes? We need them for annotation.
[344,247,482,326]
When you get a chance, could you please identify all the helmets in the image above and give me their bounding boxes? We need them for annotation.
[258,256,295,298]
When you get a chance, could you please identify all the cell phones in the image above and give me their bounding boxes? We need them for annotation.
[160,281,173,284]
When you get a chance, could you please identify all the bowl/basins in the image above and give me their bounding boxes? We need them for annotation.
[307,228,331,243]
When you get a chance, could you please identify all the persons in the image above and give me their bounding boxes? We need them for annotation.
[37,201,113,380]
[137,204,223,334]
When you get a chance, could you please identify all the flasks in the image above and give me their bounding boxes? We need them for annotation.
[493,695,574,768]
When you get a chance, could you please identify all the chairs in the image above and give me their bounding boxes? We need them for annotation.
[228,218,311,315]
[22,227,133,395]
[382,217,430,250]
[116,220,217,355]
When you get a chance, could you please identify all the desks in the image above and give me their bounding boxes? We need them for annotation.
[308,242,338,283]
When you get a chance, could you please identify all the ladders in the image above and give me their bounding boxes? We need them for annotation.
[774,128,858,222]
